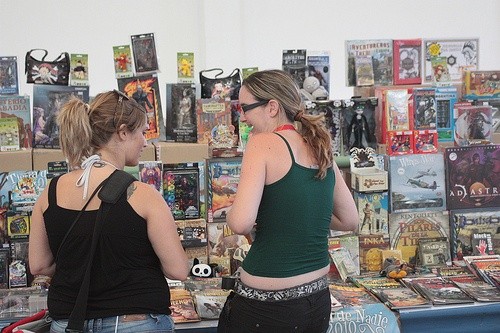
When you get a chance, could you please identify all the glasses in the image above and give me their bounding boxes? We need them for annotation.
[241,100,267,113]
[112,88,129,129]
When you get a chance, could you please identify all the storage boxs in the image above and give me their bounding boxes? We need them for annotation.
[0,34,249,291]
[304,38,500,275]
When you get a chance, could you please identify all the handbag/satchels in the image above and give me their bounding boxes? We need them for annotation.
[22,50,71,87]
[198,68,241,101]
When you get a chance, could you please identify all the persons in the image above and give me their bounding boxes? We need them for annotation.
[178,89,192,130]
[347,104,370,151]
[132,86,153,130]
[29,91,188,333]
[219,70,358,333]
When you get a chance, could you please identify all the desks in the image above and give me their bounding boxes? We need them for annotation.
[172,301,500,333]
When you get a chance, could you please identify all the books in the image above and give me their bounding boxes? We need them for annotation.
[417,238,452,266]
[402,274,475,305]
[464,254,500,289]
[472,233,493,255]
[429,264,500,302]
[162,274,201,323]
[186,276,234,320]
[348,272,433,309]
[327,278,389,312]
